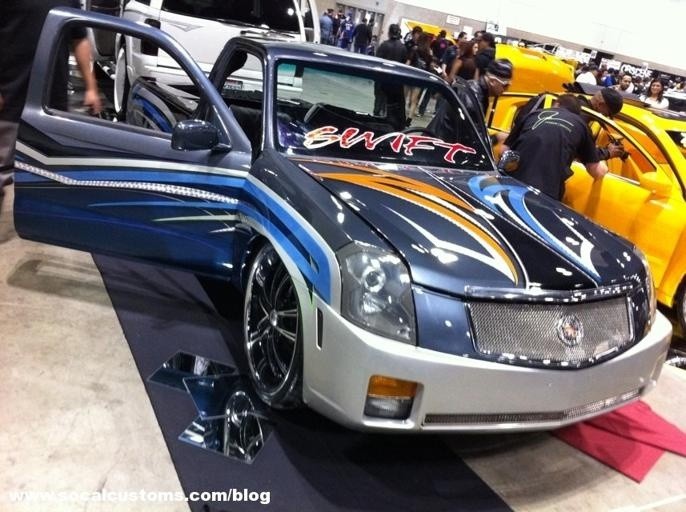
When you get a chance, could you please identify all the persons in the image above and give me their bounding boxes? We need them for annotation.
[0,1,102,212]
[319,9,686,202]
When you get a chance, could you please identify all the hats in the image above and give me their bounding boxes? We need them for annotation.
[486,56,515,82]
[599,87,625,120]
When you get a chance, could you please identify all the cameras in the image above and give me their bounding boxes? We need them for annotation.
[606,138,630,162]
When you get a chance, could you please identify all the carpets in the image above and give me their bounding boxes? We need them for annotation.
[91,249,511,512]
